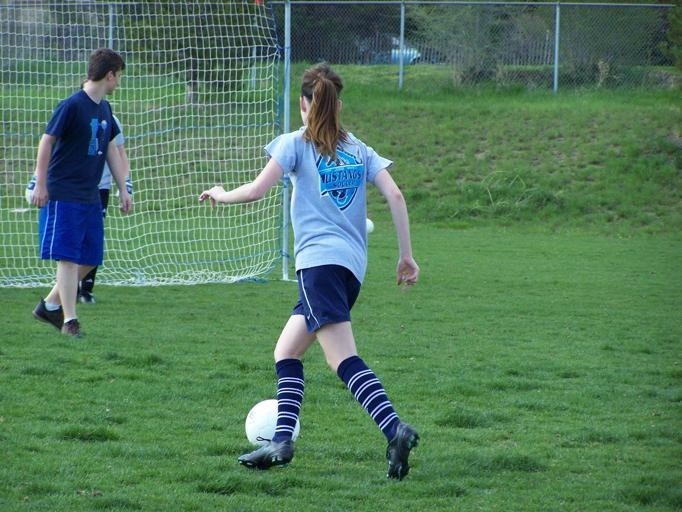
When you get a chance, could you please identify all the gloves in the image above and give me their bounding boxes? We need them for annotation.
[25,181,35,204]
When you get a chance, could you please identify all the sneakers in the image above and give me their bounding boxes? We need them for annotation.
[236,435,294,469]
[385,425,422,480]
[80,292,96,305]
[31,294,64,332]
[60,316,85,340]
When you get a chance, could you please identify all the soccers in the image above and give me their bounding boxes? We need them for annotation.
[366,218,375,234]
[245,398,300,446]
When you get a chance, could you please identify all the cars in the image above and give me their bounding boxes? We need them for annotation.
[361,33,420,66]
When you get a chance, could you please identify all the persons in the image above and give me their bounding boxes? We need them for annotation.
[199,63,421,478]
[24,48,135,337]
